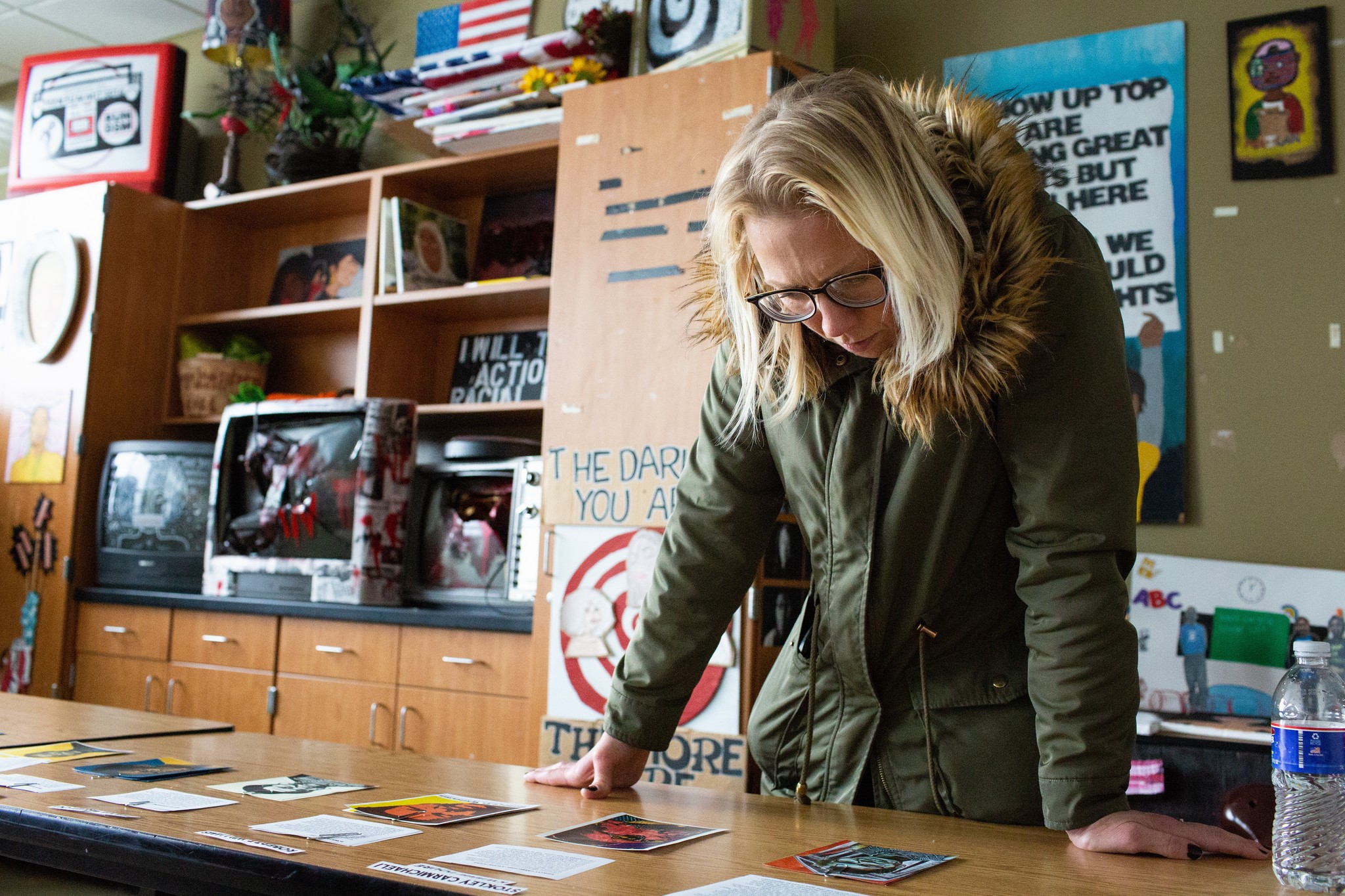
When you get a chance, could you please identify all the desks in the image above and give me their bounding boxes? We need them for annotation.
[0,684,243,761]
[4,723,1345,896]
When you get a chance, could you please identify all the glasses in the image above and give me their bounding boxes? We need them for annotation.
[743,262,888,323]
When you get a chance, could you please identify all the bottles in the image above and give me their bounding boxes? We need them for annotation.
[1271,639,1345,895]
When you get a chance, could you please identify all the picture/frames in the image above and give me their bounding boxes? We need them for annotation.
[1222,6,1339,183]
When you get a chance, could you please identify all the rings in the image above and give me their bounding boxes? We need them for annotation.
[1179,819,1185,822]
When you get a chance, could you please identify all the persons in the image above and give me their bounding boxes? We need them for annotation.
[242,775,372,794]
[313,248,359,300]
[525,62,1275,861]
[1321,615,1345,720]
[439,508,504,588]
[1286,617,1323,719]
[822,845,947,875]
[1180,605,1207,709]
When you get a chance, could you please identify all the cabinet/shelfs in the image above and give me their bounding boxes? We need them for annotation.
[0,176,177,706]
[69,586,540,779]
[165,129,561,428]
[522,52,822,789]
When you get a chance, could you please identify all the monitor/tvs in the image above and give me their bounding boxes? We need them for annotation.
[203,401,424,606]
[97,438,216,593]
[400,455,542,607]
